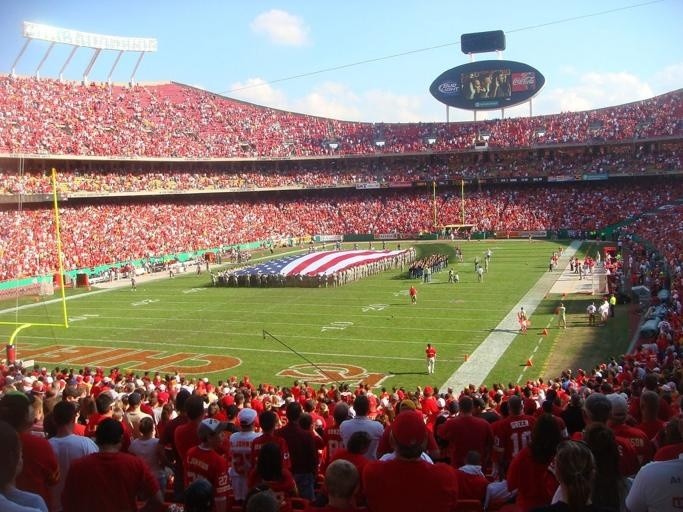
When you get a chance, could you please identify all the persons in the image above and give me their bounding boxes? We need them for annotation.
[1,72,682,512]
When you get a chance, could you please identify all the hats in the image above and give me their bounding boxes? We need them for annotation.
[390,410,430,449]
[0,390,36,409]
[435,398,446,408]
[664,381,676,390]
[605,393,627,420]
[67,379,77,386]
[127,392,142,405]
[235,407,257,426]
[423,384,434,396]
[398,400,417,412]
[156,393,170,404]
[197,416,228,441]
[30,384,46,394]
[459,395,474,409]
[659,385,671,392]
